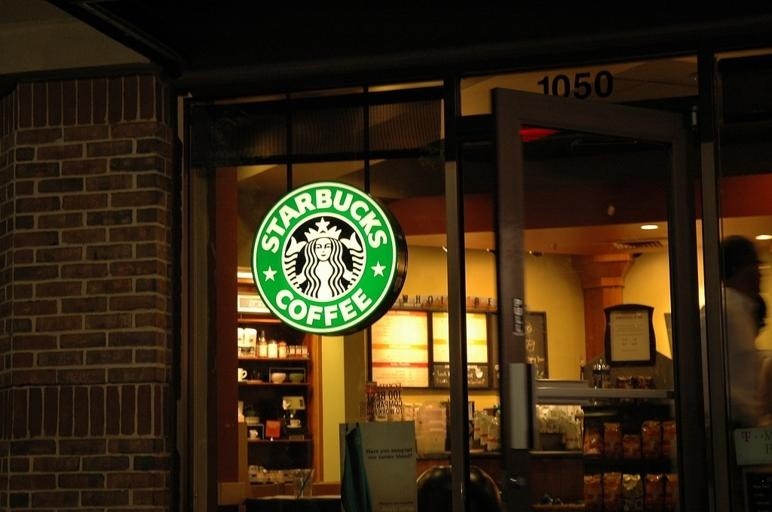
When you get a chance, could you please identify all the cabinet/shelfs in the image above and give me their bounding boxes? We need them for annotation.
[235,279,322,494]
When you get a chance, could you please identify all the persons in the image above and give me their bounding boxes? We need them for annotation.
[689,234,767,458]
